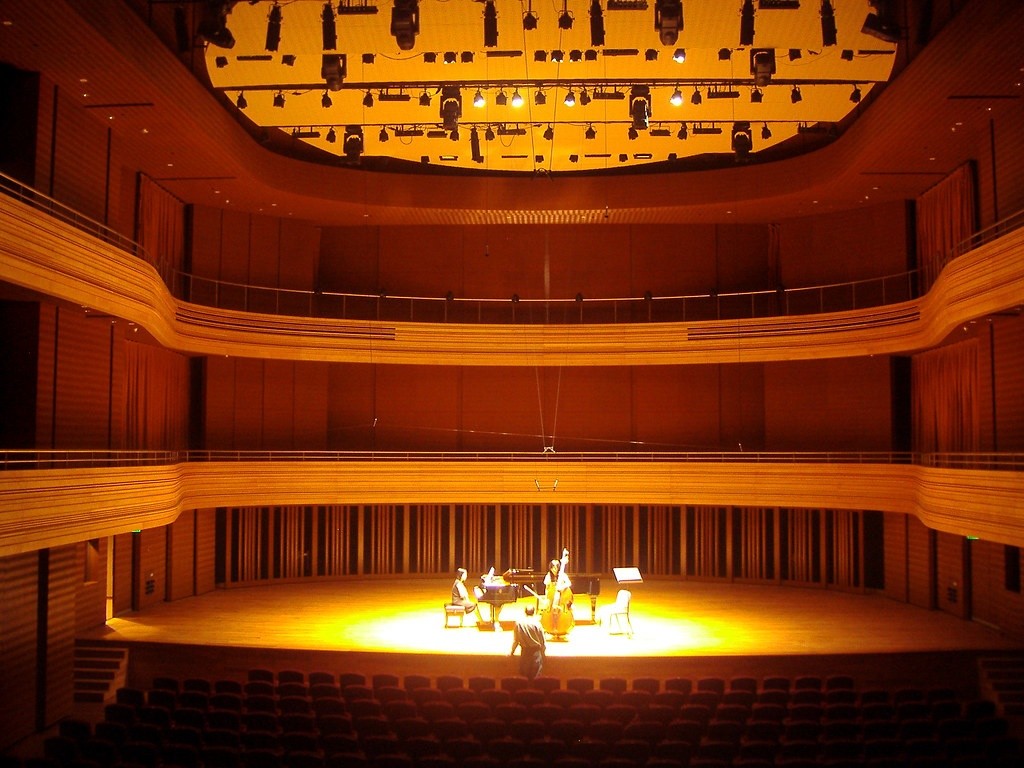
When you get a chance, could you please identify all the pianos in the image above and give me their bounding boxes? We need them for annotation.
[476,569,601,631]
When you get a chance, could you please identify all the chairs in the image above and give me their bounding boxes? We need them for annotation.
[27,669,1024,768]
[599,588,631,634]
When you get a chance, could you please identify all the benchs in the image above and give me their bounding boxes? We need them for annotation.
[445,605,466,629]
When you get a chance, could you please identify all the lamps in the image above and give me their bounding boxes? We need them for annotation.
[343,125,364,166]
[473,85,486,108]
[512,87,524,108]
[390,0,420,50]
[440,87,462,130]
[749,48,776,87]
[321,54,347,92]
[670,88,683,106]
[628,85,651,130]
[672,48,686,64]
[731,121,753,164]
[563,88,576,107]
[654,0,684,47]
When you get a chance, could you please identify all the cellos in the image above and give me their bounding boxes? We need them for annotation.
[540,548,575,635]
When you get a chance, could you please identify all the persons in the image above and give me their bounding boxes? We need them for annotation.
[452,568,488,626]
[544,559,572,591]
[511,605,546,682]
